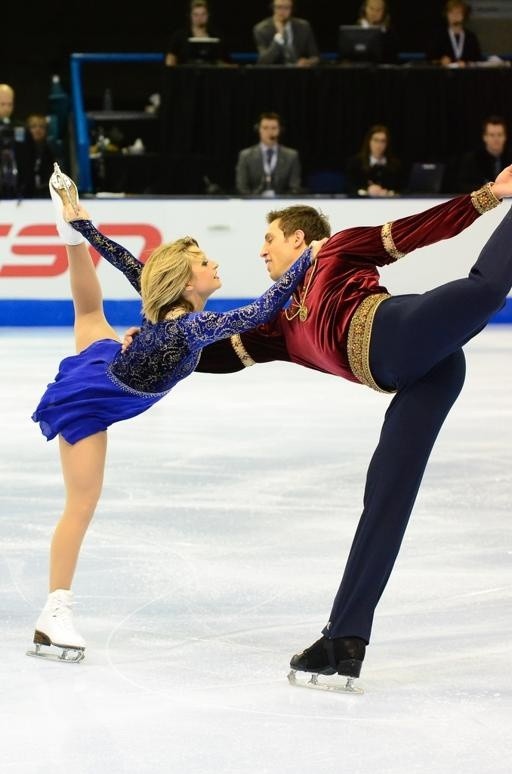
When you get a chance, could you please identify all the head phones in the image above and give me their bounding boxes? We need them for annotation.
[253,122,286,132]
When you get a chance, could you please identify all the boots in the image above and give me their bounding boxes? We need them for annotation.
[34,590,85,649]
[48,172,92,246]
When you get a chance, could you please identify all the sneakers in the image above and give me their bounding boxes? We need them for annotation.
[291,635,364,677]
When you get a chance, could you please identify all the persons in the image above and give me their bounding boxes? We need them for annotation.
[118,165,512,691]
[233,112,301,195]
[251,0,321,66]
[20,114,68,197]
[93,130,152,160]
[463,113,511,190]
[26,164,329,662]
[426,0,489,68]
[1,84,18,197]
[356,0,399,63]
[344,124,408,198]
[165,0,235,66]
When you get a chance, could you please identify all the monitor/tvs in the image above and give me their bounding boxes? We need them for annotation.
[338,24,383,64]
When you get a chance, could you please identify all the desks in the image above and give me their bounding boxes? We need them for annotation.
[149,60,511,167]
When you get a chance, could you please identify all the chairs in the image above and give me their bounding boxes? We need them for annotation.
[311,175,344,194]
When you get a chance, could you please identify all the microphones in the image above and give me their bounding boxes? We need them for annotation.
[270,135,282,139]
